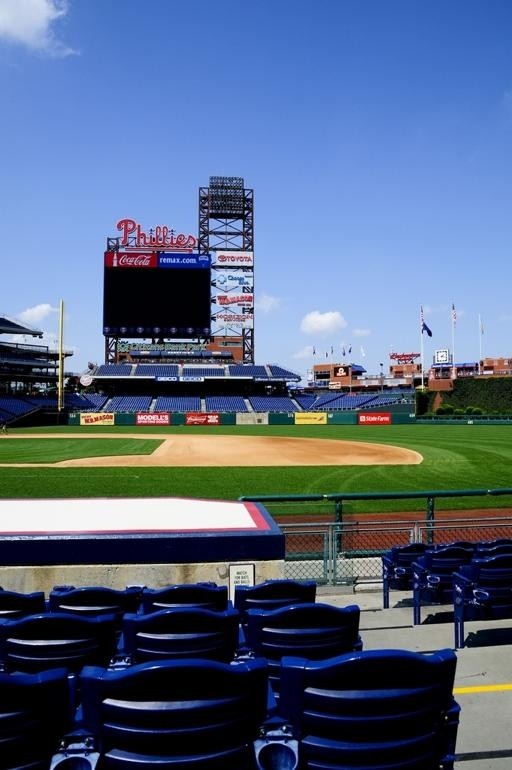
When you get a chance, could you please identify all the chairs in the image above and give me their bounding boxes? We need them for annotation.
[0,577,461,770]
[1,390,71,427]
[381,537,512,649]
[72,364,416,412]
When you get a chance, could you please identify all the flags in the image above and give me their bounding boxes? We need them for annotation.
[452,304,457,327]
[479,317,485,335]
[420,304,432,338]
[312,342,368,359]
